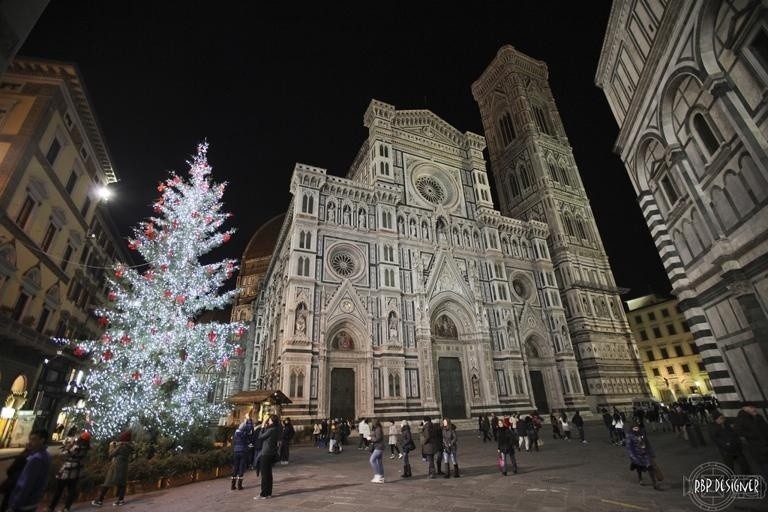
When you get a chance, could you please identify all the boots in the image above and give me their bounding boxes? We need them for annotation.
[429,462,459,478]
[402,464,411,477]
[231,477,243,490]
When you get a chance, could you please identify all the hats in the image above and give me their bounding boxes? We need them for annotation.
[81,431,91,440]
[120,431,132,440]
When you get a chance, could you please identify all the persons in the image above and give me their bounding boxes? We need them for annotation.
[0,430,90,512]
[91,429,134,507]
[229,394,768,502]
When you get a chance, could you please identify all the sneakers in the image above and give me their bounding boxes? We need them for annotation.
[113,499,125,506]
[254,494,266,500]
[372,474,384,484]
[91,499,102,506]
